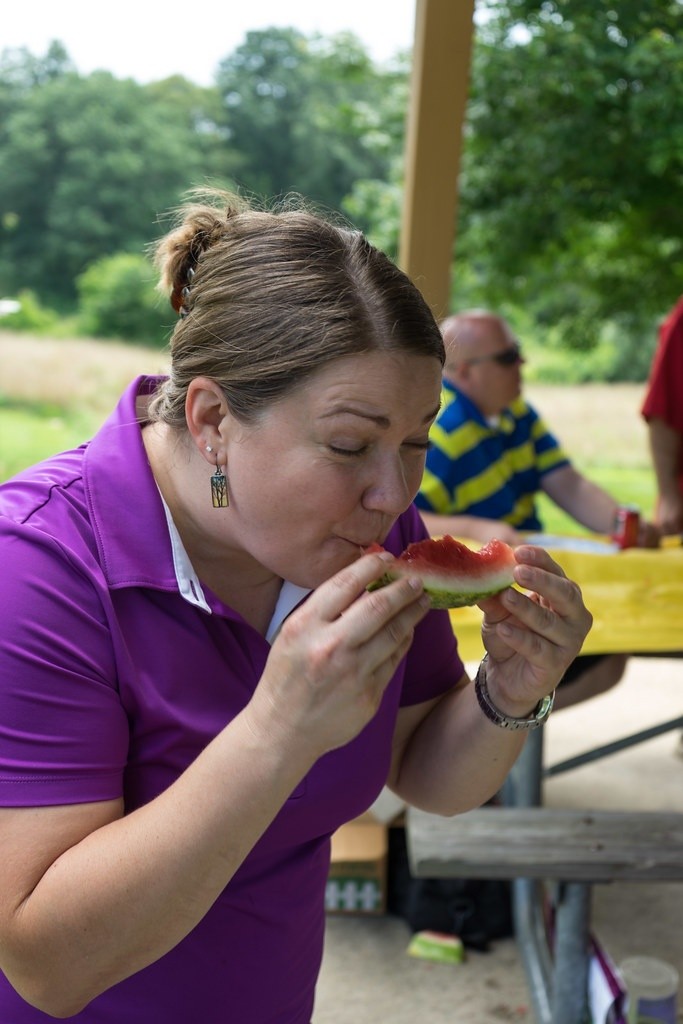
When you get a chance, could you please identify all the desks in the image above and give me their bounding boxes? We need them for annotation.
[428,533,683,1024]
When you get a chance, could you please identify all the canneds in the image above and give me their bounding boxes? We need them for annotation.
[610,504,641,549]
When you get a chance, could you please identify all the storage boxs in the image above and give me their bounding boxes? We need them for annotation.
[322,784,406,917]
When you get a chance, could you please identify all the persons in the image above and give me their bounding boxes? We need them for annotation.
[1,180,595,1024]
[411,304,662,553]
[639,306,683,547]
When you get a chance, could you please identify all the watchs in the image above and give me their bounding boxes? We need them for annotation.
[475,652,558,730]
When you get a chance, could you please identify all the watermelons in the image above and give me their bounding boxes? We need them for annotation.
[361,536,517,608]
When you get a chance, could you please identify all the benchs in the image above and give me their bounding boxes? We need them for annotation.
[407,807,683,884]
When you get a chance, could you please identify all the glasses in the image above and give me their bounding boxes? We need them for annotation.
[447,342,520,370]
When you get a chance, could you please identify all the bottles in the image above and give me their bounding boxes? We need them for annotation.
[607,955,680,1024]
